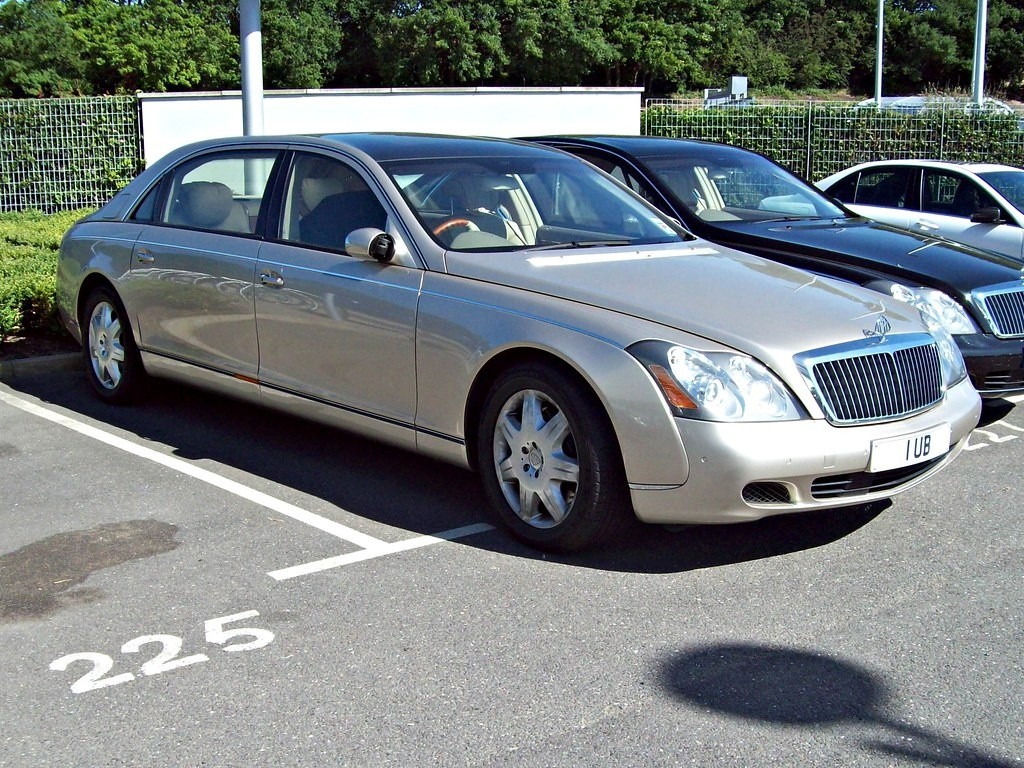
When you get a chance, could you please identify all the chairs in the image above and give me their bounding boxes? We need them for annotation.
[171,181,251,233]
[954,181,979,211]
[658,171,707,216]
[301,177,344,211]
[434,175,525,245]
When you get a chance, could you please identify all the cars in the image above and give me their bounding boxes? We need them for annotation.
[56,130,983,553]
[759,157,1024,265]
[399,134,1024,423]
